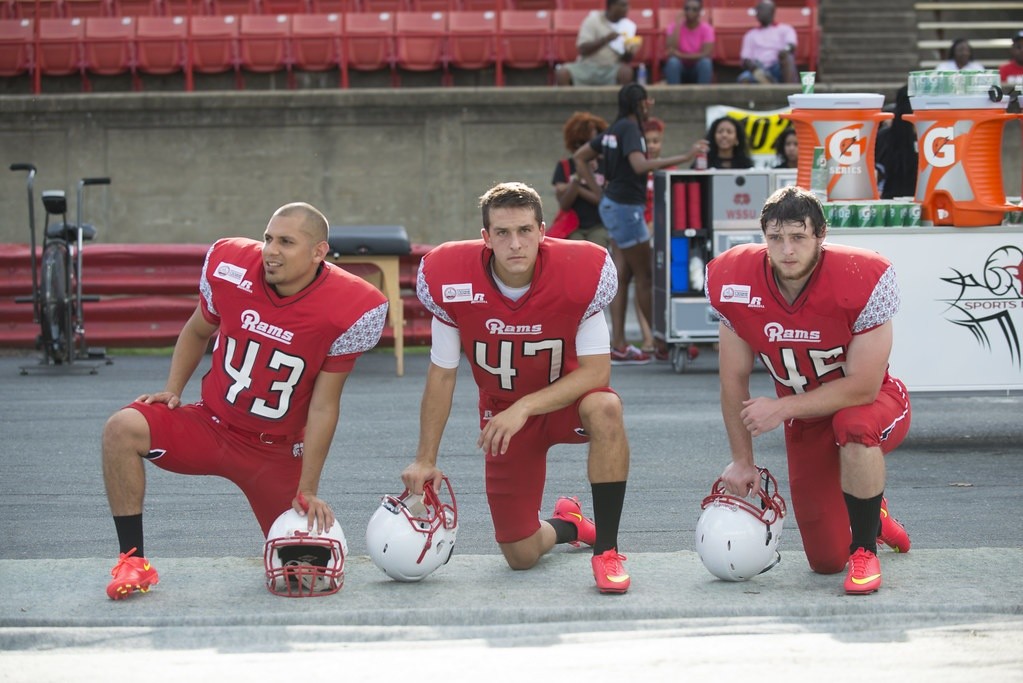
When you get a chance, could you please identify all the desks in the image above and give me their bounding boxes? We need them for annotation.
[824,223,1023,394]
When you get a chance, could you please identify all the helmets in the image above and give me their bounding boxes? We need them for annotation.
[366,475,459,582]
[263,508,349,597]
[695,466,786,582]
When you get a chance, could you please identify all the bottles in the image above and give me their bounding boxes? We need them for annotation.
[637,63,647,85]
[695,149,708,170]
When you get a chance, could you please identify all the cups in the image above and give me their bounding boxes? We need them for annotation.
[820,200,922,226]
[800,72,816,94]
[813,147,825,169]
[907,71,1001,96]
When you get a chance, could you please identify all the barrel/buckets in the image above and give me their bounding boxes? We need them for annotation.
[901,97,1023,227]
[778,93,894,205]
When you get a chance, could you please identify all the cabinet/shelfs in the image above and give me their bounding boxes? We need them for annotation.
[653,167,797,371]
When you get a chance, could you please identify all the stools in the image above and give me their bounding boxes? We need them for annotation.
[324,225,412,376]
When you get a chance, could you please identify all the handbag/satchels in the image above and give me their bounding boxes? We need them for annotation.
[545,160,579,239]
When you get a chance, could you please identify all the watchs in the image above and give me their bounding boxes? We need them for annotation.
[785,45,793,53]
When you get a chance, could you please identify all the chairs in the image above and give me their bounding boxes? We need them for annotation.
[0,0,817,99]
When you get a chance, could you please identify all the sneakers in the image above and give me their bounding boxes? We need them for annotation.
[876,498,912,554]
[553,496,596,547]
[592,547,630,593]
[106,547,159,600]
[640,346,657,362]
[844,547,882,595]
[610,345,648,365]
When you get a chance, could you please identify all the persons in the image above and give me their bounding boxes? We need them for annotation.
[733,0,798,84]
[552,0,642,87]
[883,31,1023,200]
[706,186,912,595]
[401,182,632,596]
[100,201,388,599]
[544,84,709,365]
[690,115,754,170]
[663,0,715,84]
[776,129,801,170]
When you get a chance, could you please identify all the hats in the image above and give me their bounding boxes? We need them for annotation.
[1012,30,1023,39]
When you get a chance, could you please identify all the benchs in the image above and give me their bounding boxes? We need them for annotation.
[914,1,1023,67]
[0,240,434,346]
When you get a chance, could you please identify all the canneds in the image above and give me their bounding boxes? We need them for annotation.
[695,152,708,169]
